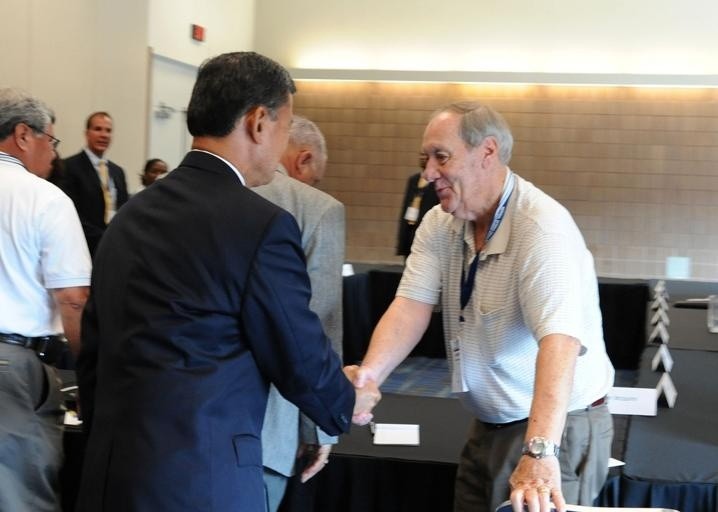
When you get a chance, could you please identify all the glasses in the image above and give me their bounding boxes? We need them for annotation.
[28,125,60,149]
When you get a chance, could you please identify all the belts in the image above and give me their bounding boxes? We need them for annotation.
[0,332,41,350]
[481,397,605,429]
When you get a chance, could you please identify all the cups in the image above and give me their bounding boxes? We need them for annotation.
[707,293,717,334]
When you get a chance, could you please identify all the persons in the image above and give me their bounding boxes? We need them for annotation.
[0,89,93,512]
[76,51,383,512]
[395,145,440,267]
[47,113,128,343]
[249,114,348,512]
[142,158,169,187]
[340,102,615,511]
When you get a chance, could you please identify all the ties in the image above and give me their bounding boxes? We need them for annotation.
[99,159,112,225]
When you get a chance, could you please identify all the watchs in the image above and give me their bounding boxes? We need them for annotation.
[522,435,559,460]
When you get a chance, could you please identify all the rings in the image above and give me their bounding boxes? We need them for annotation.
[536,489,549,496]
[324,459,330,464]
